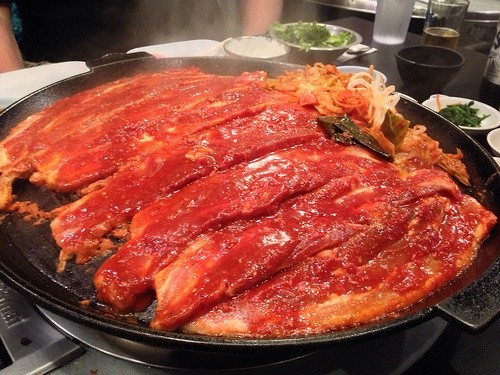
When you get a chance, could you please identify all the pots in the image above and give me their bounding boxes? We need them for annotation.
[0,50,499,359]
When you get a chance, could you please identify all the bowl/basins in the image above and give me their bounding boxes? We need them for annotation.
[394,45,465,93]
[269,22,362,64]
[221,35,291,63]
[486,128,500,156]
[333,65,387,86]
[421,94,500,135]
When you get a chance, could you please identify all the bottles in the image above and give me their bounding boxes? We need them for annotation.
[483,18,500,86]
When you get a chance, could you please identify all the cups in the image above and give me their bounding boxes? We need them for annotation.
[420,0,470,52]
[373,0,416,45]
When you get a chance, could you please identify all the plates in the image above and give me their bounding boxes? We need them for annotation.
[126,39,223,58]
[1,61,91,113]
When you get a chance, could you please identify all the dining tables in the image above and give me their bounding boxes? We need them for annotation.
[257,16,500,375]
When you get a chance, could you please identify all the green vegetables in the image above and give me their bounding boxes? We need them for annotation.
[436,101,492,127]
[272,19,354,52]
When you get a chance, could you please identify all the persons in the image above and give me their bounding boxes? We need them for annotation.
[0,0,283,76]
